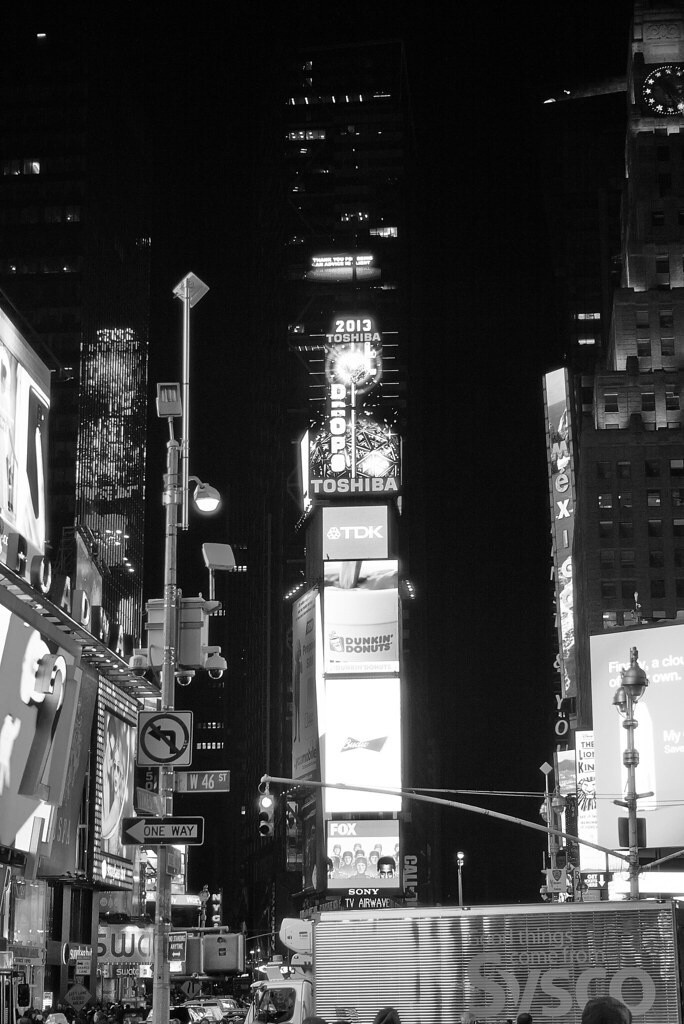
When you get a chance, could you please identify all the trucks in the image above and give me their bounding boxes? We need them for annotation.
[244,896,684,1024]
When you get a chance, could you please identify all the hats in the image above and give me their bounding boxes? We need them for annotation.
[581,996,632,1024]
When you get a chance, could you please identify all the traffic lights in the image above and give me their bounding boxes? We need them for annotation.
[121,816,205,846]
[259,794,275,836]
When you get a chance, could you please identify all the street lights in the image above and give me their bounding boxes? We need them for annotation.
[540,785,567,903]
[611,646,651,899]
[456,851,465,905]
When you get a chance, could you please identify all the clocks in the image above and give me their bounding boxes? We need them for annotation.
[642,65,684,116]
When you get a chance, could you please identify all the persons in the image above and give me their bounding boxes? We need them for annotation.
[200,1018,234,1024]
[16,1003,153,1024]
[517,1013,532,1024]
[328,857,334,879]
[302,1017,326,1024]
[103,716,128,855]
[582,997,632,1024]
[172,1019,180,1024]
[375,857,396,877]
